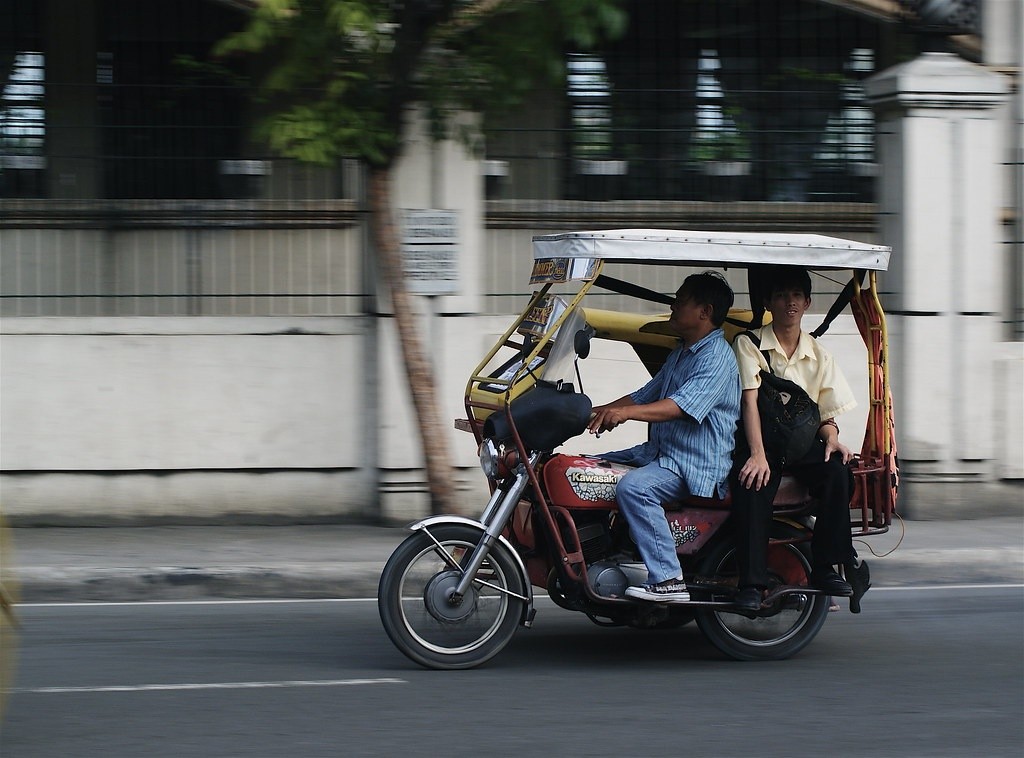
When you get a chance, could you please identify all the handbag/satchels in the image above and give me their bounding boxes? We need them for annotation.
[758,369,821,459]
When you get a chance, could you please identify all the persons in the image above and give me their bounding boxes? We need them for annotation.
[734,266,855,600]
[590,270,743,604]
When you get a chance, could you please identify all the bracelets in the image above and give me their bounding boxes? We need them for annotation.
[823,420,839,433]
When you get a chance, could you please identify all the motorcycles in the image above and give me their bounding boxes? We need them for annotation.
[373,224,903,672]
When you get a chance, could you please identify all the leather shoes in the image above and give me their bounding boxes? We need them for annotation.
[736,587,761,611]
[809,571,853,596]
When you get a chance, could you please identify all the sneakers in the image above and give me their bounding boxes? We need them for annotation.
[625,578,690,601]
